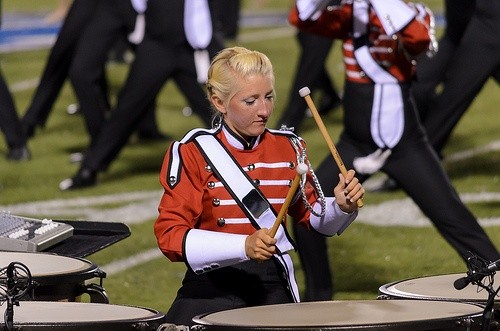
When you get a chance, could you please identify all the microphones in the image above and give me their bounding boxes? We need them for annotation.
[453,260,500,291]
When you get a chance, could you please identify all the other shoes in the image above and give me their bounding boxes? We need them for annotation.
[66,103,81,116]
[138,131,171,145]
[307,95,341,119]
[59,174,98,190]
[68,147,87,162]
[7,144,29,161]
[383,177,399,191]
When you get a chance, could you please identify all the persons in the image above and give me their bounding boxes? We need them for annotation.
[274,0,500,301]
[0,0,242,191]
[154,47,366,325]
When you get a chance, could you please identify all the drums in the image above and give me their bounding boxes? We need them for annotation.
[0,249,106,301]
[378,268,500,331]
[187,299,486,331]
[0,299,167,331]
